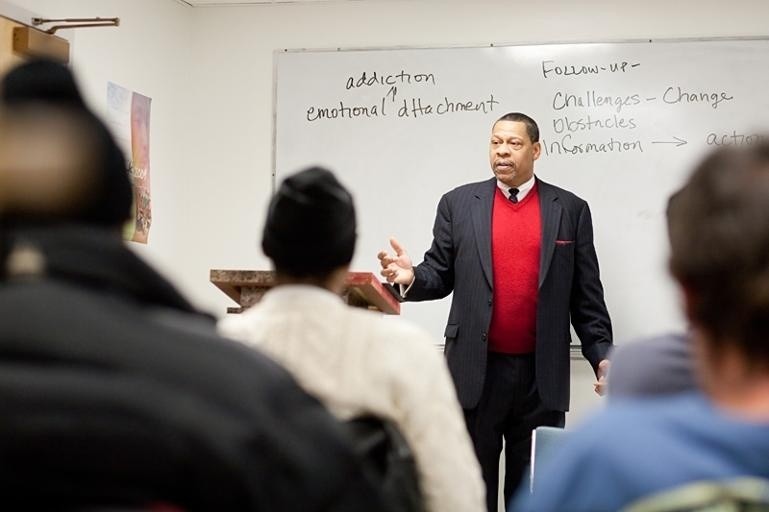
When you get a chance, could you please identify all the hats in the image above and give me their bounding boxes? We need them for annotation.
[0,54,135,231]
[262,166,356,272]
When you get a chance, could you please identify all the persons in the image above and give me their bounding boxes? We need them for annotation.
[507,129,768,511]
[378,112,615,511]
[2,58,490,510]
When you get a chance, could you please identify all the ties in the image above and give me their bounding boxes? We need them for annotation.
[508,186,519,204]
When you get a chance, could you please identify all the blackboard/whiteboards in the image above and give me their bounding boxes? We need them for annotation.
[271,35,769,359]
